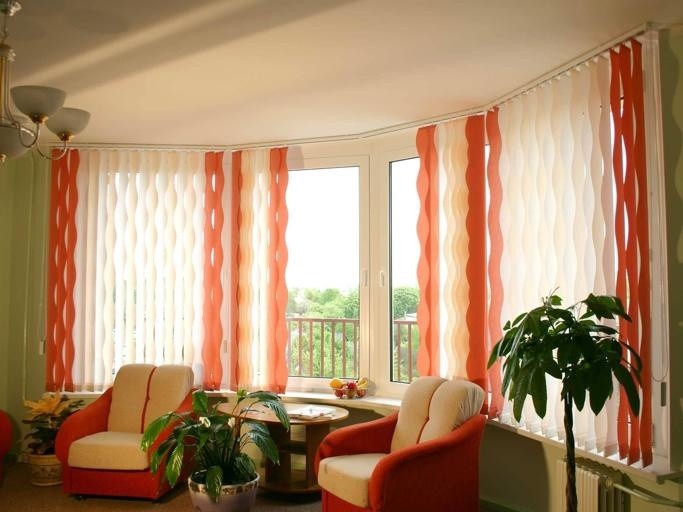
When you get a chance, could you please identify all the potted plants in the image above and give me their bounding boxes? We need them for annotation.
[140,389,291,512]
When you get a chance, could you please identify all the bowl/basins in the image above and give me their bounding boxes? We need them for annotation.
[331,387,368,399]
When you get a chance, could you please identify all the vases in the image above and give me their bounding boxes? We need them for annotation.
[27,454,63,484]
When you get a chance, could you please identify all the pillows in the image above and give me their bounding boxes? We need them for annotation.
[106,363,193,435]
[390,376,484,455]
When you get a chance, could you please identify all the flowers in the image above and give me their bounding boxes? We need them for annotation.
[21,388,85,456]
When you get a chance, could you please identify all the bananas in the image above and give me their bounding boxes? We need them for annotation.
[357,378,368,389]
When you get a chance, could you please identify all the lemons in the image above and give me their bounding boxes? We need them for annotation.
[330,378,343,390]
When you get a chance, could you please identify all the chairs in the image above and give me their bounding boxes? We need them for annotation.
[0,410,13,486]
[54,363,200,505]
[313,375,487,512]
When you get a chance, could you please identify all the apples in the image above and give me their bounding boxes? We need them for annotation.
[342,385,349,390]
[334,390,343,396]
[347,383,358,388]
[357,390,365,397]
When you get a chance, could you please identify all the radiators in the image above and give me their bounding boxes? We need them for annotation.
[555,457,625,512]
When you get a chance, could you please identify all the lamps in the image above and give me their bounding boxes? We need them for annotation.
[0,0,91,165]
